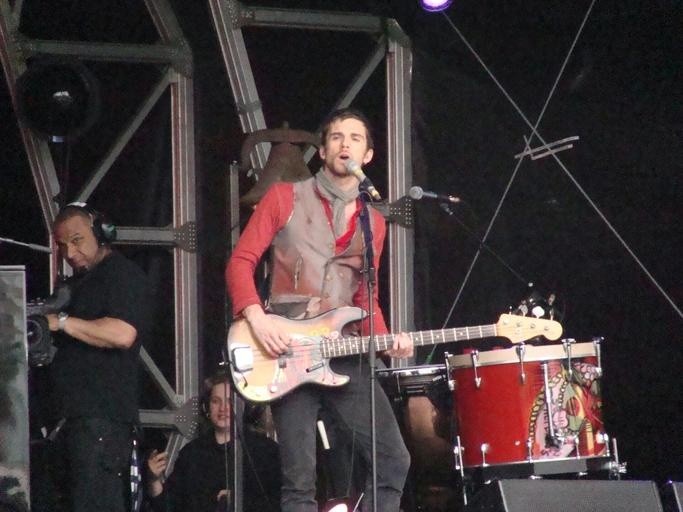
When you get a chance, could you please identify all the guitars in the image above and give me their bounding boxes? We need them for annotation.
[227,306,563,404]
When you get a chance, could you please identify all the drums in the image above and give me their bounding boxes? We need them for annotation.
[376,364,477,511]
[444,337,614,486]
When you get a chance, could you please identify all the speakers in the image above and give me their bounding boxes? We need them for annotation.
[462,478,663,512]
[27,300,51,360]
[659,480,683,512]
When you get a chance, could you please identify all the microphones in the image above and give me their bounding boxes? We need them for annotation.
[344,158,381,202]
[409,185,460,204]
[545,295,556,319]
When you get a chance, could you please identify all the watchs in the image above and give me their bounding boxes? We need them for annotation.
[58,310,68,332]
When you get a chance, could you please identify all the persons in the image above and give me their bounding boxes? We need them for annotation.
[143,374,282,512]
[224,107,415,512]
[28,202,147,512]
[403,336,513,512]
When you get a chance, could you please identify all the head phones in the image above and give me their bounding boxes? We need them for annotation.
[67,200,118,245]
[200,374,264,423]
